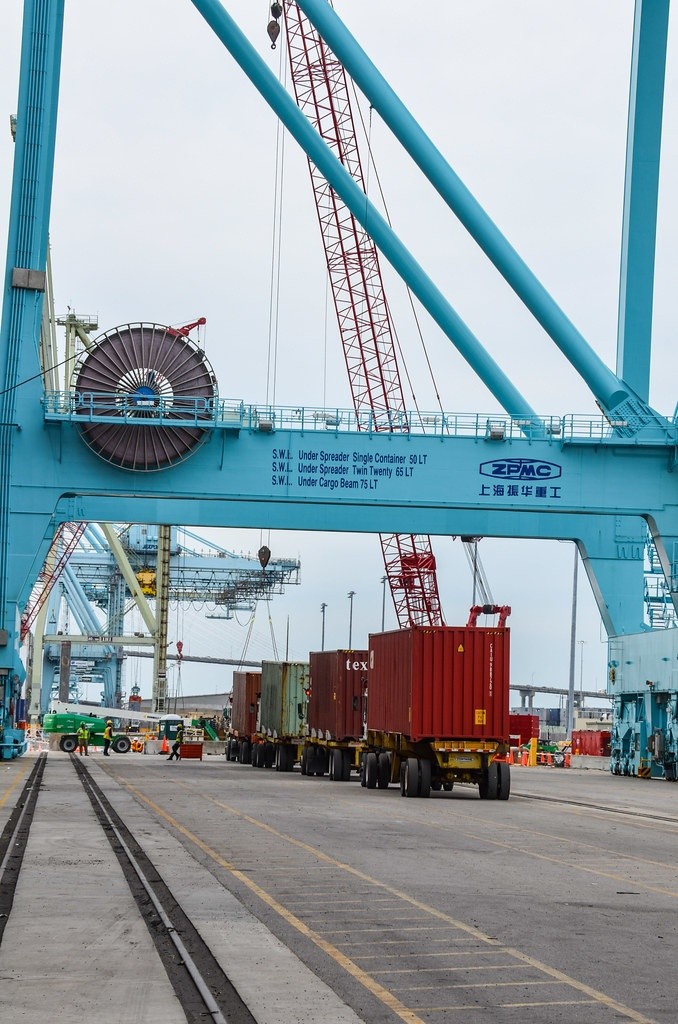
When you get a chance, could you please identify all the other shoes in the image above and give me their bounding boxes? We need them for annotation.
[167,757,172,760]
[85,754,89,756]
[81,754,82,756]
[176,754,180,760]
[104,753,110,756]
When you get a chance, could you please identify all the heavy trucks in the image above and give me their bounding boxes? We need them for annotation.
[248,660,310,772]
[223,671,263,764]
[352,604,511,800]
[297,649,367,781]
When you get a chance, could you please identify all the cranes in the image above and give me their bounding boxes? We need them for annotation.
[267,0,448,632]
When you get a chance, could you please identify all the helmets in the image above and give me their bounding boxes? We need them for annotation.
[107,720,112,724]
[134,737,138,741]
[178,724,182,729]
[80,721,86,724]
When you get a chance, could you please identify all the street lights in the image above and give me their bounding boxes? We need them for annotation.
[321,602,328,651]
[347,590,356,650]
[380,575,389,632]
[459,535,485,607]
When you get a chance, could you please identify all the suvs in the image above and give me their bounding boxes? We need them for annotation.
[43,713,132,753]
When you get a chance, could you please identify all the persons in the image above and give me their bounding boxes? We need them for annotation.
[76,721,90,756]
[130,737,143,753]
[166,724,183,761]
[103,720,113,756]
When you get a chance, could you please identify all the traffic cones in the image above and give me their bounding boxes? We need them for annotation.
[520,748,529,766]
[546,751,556,767]
[158,734,169,755]
[562,752,570,768]
[538,750,547,764]
[508,748,515,766]
